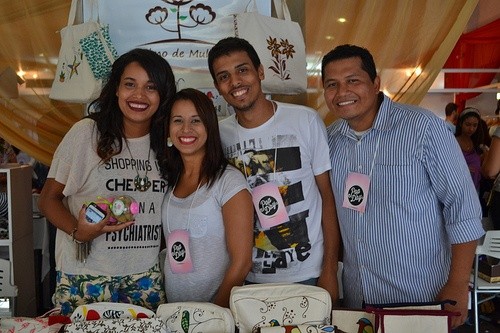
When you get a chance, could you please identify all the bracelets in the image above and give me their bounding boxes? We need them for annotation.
[70,228,89,245]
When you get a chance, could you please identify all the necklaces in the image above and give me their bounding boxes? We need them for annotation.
[122,134,152,192]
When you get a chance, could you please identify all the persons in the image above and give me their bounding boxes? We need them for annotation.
[445,99,500,228]
[320,43,485,331]
[0,137,50,223]
[208,37,340,302]
[160,88,254,309]
[39,48,176,318]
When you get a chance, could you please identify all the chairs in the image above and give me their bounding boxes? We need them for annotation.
[0,259,19,317]
[469,230,500,333]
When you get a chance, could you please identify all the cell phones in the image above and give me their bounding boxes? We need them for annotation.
[85,202,107,224]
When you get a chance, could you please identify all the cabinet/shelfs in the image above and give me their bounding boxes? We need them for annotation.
[0,162,37,317]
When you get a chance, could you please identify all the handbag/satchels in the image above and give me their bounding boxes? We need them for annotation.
[0,282,461,333]
[229,0,307,95]
[49,0,120,104]
